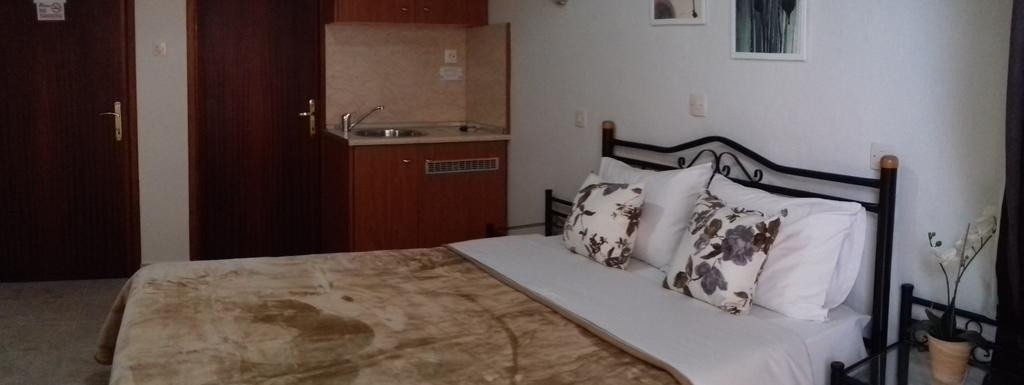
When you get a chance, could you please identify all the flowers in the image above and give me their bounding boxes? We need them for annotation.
[920,203,1003,340]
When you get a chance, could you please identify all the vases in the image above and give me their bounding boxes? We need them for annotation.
[927,335,975,382]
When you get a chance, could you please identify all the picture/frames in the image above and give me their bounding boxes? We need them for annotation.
[730,0,808,61]
[649,0,705,26]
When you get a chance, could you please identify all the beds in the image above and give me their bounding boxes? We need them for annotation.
[92,120,897,385]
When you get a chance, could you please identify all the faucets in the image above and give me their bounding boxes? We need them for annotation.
[341,105,384,132]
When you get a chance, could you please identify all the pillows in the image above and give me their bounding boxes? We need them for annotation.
[562,170,646,277]
[664,189,781,319]
[566,160,715,279]
[674,174,864,325]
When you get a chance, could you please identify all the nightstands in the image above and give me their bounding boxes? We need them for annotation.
[831,282,1004,385]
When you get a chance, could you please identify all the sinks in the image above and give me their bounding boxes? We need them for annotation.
[353,129,428,137]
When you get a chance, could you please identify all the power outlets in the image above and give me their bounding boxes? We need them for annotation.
[443,48,463,65]
